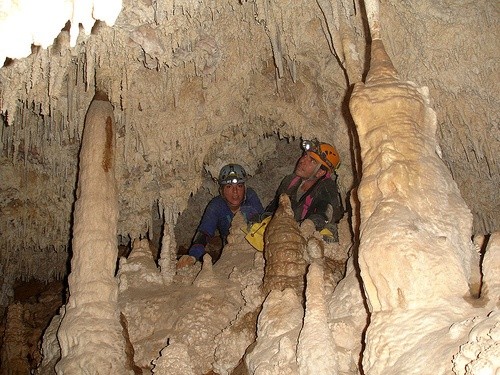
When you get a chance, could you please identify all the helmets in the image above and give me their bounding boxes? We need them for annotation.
[306,143,341,172]
[218,164,247,185]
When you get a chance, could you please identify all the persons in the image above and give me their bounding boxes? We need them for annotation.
[176,164,267,269]
[261,138,346,242]
